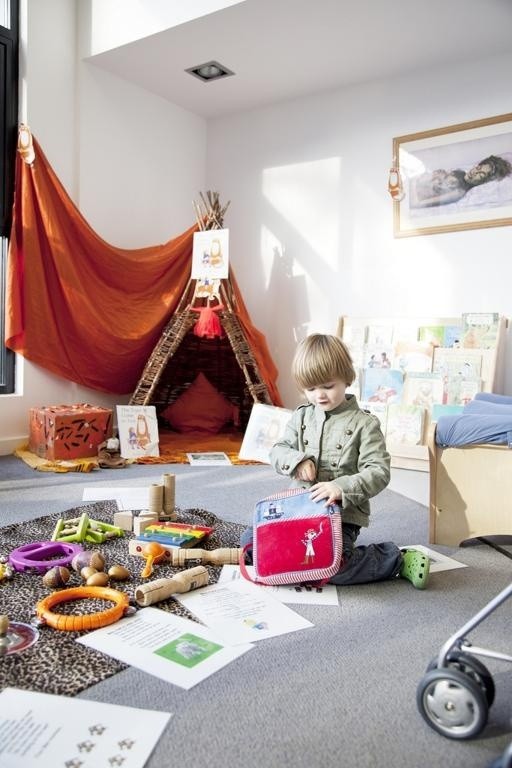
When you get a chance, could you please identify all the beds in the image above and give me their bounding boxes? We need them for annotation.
[428,392,512,548]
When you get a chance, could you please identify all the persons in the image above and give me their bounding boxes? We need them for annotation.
[137,414,151,449]
[269,334,430,591]
[128,427,137,449]
[209,240,223,268]
[408,154,510,212]
[202,250,210,267]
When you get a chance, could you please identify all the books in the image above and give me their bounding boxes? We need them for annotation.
[342,312,501,448]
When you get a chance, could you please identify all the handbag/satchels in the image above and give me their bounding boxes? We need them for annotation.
[239,483,342,588]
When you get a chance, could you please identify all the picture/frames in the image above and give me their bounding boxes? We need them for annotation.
[392,112,512,240]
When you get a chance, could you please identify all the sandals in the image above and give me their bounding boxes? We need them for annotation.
[398,548,430,590]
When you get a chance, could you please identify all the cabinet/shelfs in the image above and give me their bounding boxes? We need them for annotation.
[333,312,507,473]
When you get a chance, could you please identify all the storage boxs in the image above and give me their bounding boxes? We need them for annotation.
[29,402,116,462]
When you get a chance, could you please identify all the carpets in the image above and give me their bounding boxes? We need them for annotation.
[0,500,252,697]
